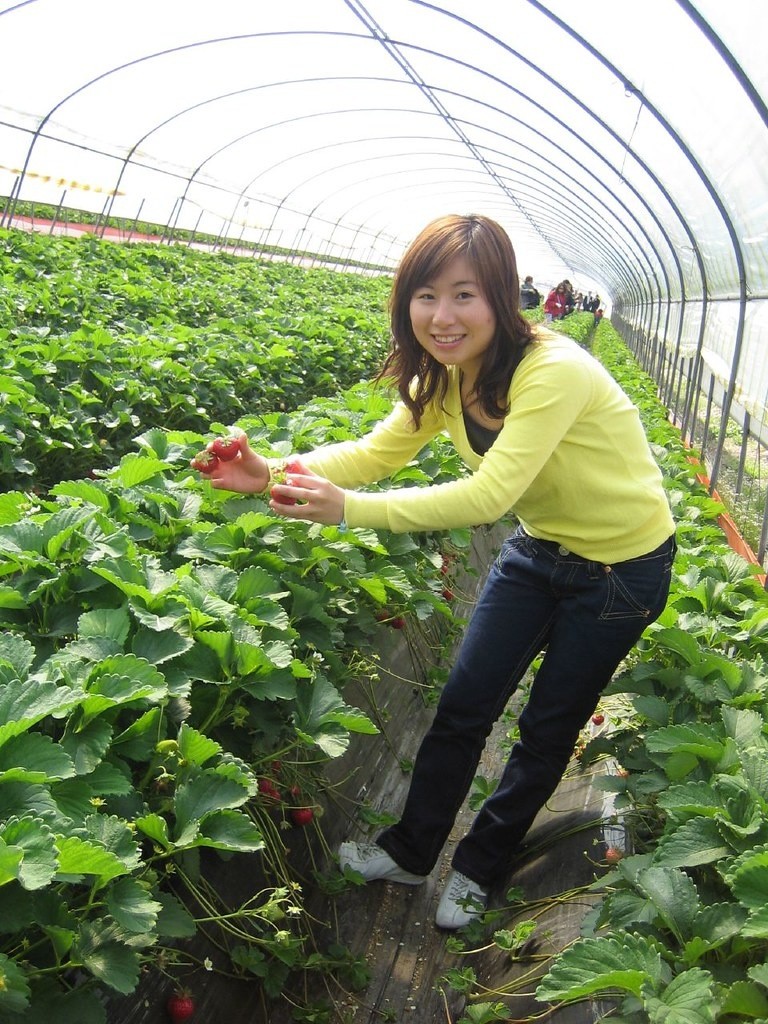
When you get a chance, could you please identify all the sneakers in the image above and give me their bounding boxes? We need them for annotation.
[434,866,489,929]
[337,840,427,885]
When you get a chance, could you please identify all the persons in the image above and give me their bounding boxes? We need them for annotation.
[520,276,540,310]
[190,214,675,929]
[545,280,604,327]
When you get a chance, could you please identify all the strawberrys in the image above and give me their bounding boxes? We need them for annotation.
[166,994,194,1024]
[253,555,625,869]
[195,434,305,505]
